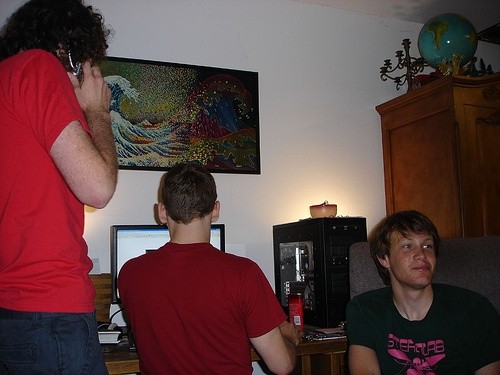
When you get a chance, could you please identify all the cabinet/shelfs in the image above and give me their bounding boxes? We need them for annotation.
[375,75,500,238]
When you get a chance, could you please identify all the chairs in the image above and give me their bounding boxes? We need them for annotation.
[347,238,500,305]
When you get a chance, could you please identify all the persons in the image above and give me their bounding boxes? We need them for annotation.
[347,210,500,375]
[0,0,117,375]
[119,160,303,375]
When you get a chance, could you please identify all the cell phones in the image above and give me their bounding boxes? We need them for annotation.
[67,47,84,87]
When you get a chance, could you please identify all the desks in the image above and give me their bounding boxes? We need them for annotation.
[93,325,347,375]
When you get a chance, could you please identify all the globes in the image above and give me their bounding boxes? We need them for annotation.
[417,13,477,77]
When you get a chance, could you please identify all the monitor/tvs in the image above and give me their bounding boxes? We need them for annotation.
[111,224,225,305]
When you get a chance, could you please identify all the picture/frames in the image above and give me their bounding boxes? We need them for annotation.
[79,56,261,175]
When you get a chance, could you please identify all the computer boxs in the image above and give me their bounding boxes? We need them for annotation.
[273,217,367,329]
